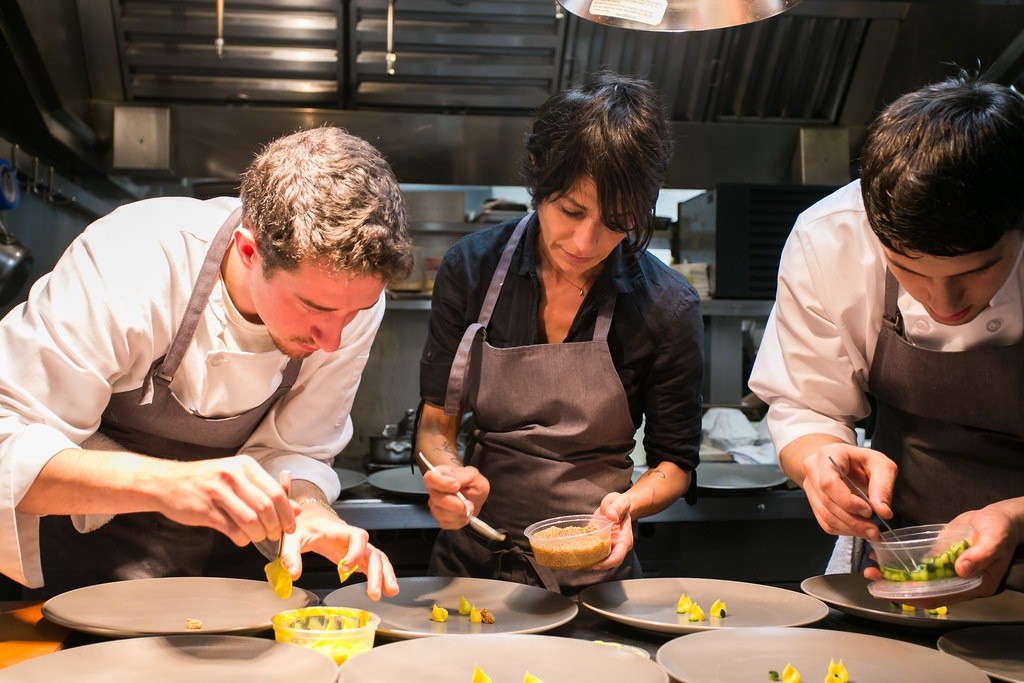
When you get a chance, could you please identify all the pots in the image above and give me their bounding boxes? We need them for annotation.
[366,408,415,461]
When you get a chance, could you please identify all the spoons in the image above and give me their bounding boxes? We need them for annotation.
[418,451,507,541]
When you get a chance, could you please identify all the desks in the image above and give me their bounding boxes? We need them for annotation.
[0,600,1024,683]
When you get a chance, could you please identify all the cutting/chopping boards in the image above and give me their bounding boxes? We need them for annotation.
[700,441,731,461]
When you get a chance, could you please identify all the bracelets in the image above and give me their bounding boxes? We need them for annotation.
[297,497,338,517]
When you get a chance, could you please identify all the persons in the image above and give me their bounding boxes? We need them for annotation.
[747,81,1023,576]
[1,126,407,601]
[416,76,706,592]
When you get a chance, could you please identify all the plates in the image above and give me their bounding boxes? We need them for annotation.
[332,467,367,491]
[322,577,579,638]
[656,627,991,683]
[41,577,320,634]
[0,635,339,683]
[937,625,1024,683]
[579,577,829,634]
[694,462,788,489]
[338,634,670,683]
[800,573,1024,625]
[367,465,430,494]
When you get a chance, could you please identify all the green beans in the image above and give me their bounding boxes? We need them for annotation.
[882,540,971,580]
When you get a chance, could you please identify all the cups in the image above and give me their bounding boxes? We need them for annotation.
[388,247,424,293]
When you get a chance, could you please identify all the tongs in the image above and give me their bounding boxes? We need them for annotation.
[828,456,919,575]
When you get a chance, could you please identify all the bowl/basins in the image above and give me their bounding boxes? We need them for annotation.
[866,523,976,581]
[271,606,381,666]
[523,514,614,569]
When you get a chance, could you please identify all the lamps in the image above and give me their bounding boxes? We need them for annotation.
[558,0,801,34]
